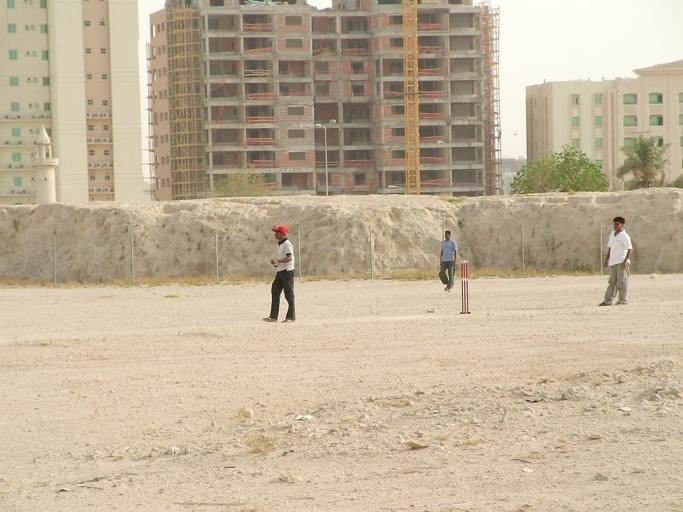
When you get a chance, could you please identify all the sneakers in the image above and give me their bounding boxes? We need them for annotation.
[264,317,277,322]
[615,301,624,305]
[281,318,293,322]
[599,302,611,306]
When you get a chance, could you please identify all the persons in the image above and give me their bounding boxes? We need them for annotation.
[261,223,297,324]
[438,229,458,293]
[597,215,635,307]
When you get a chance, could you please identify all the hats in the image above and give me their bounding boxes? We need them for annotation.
[273,226,289,236]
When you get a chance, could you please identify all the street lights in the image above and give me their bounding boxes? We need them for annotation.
[313,118,336,196]
[387,184,408,195]
